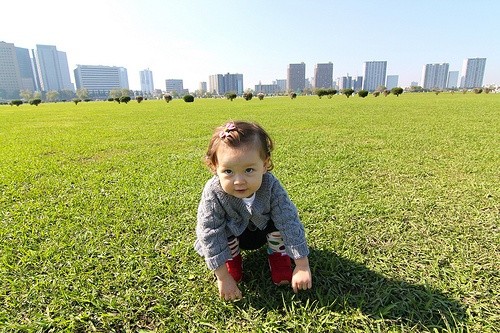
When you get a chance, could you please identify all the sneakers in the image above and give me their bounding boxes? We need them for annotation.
[267,251,293,286]
[225,254,243,283]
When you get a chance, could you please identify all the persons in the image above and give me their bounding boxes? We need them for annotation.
[194,122,313,302]
